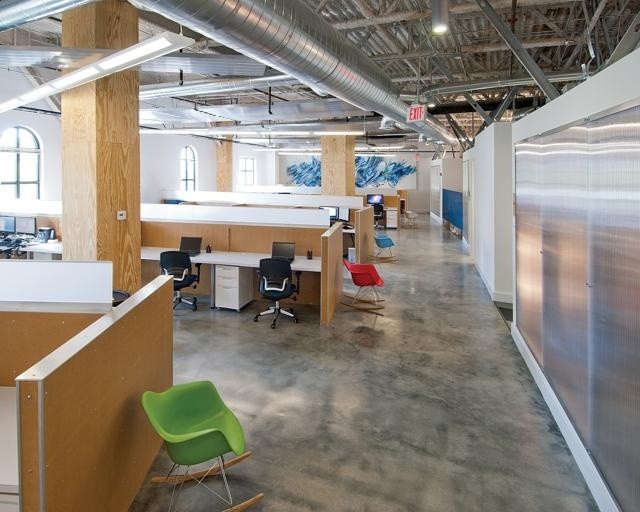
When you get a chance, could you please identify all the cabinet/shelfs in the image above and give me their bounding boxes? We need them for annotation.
[385,210,398,228]
[216,265,254,313]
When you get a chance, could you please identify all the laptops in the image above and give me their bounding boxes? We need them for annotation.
[271,242,296,264]
[179,236,202,257]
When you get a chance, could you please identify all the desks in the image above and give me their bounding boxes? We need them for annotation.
[0,233,322,309]
[341,227,359,264]
[383,207,400,230]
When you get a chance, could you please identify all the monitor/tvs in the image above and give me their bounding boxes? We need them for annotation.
[366,193,384,206]
[15,216,36,235]
[0,216,16,239]
[319,206,340,220]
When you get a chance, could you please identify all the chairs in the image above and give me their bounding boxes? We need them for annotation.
[402,208,418,226]
[139,379,264,512]
[368,233,397,259]
[371,204,384,231]
[339,258,385,312]
[254,258,298,329]
[159,250,198,312]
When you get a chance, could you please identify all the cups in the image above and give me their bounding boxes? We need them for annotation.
[206,244,212,253]
[306,250,312,259]
